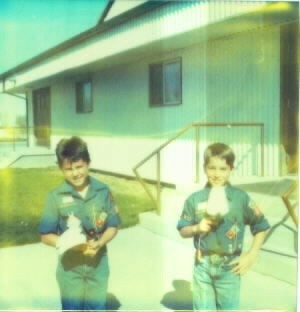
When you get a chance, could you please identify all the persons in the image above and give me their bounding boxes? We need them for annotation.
[39,136,121,312]
[177,143,269,311]
